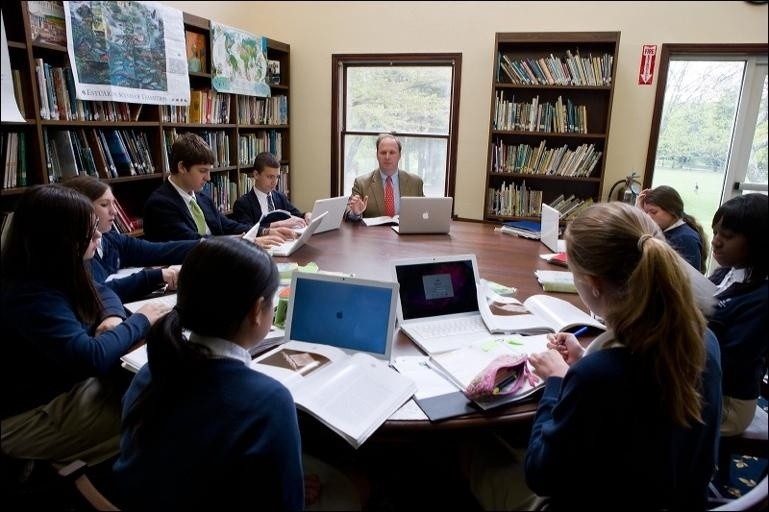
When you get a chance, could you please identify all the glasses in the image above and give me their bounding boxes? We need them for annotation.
[90,215,99,237]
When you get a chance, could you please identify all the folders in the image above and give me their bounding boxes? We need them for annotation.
[501,220,563,240]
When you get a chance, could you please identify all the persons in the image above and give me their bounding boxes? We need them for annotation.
[704,193,769,435]
[143,133,296,249]
[522,201,724,512]
[0,183,171,460]
[634,185,709,275]
[72,176,203,300]
[343,133,424,223]
[113,238,306,512]
[233,152,312,229]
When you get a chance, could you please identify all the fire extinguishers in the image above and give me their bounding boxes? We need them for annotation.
[607,173,642,206]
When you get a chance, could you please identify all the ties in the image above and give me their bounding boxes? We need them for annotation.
[385,176,395,218]
[267,194,274,213]
[190,197,206,236]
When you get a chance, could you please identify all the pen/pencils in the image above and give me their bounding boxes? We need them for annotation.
[495,371,520,392]
[548,326,589,349]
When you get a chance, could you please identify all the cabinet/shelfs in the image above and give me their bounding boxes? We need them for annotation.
[488,32,619,223]
[0,0,289,248]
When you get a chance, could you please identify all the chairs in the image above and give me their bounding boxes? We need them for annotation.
[52,458,123,511]
[685,477,769,511]
[713,365,769,484]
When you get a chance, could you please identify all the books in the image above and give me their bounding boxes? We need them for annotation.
[250,339,418,450]
[487,180,593,220]
[239,129,282,166]
[2,130,29,187]
[474,281,607,336]
[490,137,602,178]
[163,129,229,173]
[162,87,288,126]
[44,128,154,182]
[500,49,613,87]
[241,170,288,198]
[493,90,588,135]
[427,332,547,411]
[204,175,227,213]
[38,57,143,123]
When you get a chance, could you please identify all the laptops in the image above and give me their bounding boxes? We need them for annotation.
[390,196,453,235]
[291,194,349,237]
[262,209,330,257]
[390,253,522,356]
[283,269,400,363]
[540,202,569,255]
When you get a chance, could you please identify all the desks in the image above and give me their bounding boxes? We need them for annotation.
[108,215,631,437]
